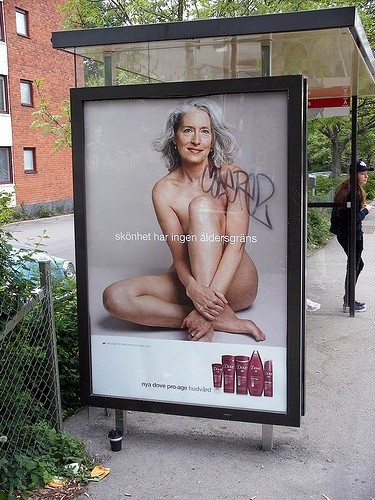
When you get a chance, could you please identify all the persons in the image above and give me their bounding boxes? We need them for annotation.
[328,161,375,313]
[101,98,266,344]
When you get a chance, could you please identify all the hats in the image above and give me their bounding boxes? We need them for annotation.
[349,160,374,175]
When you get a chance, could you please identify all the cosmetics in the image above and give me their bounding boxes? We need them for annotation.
[212,351,273,397]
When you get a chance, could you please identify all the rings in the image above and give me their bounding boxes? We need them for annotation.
[190,333,194,337]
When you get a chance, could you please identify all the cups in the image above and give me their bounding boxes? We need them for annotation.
[108,429,122,451]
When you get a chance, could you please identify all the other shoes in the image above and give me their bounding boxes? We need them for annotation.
[343,302,366,313]
